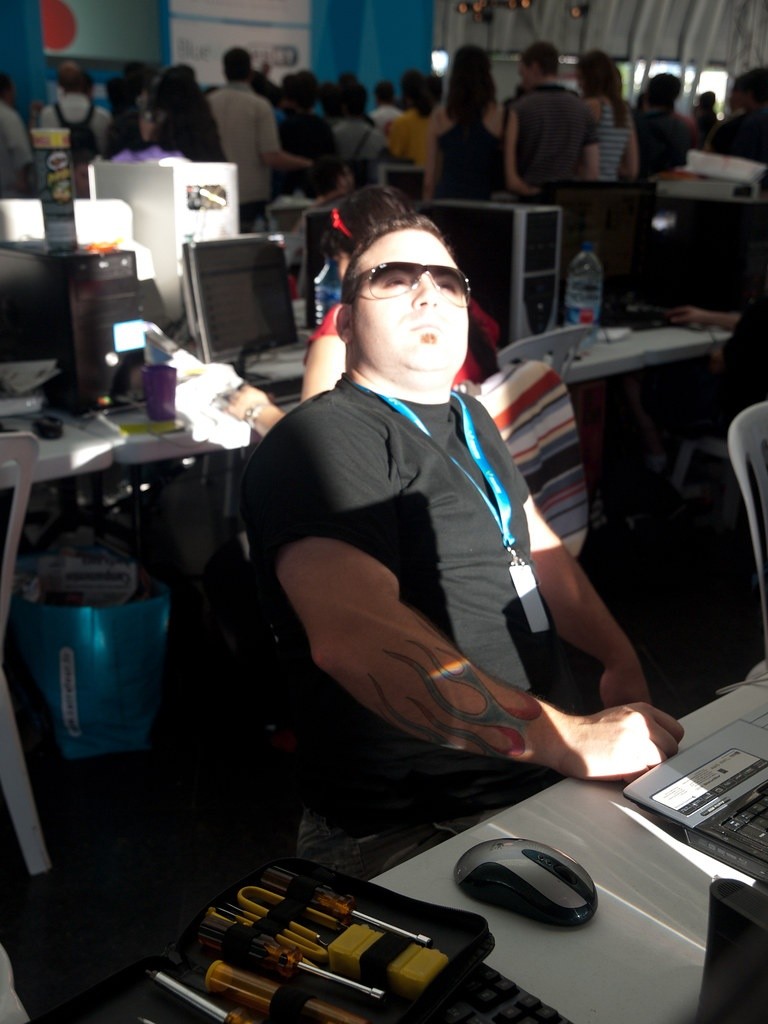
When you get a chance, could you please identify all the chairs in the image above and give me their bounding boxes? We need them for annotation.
[495,322,590,377]
[674,399,768,686]
[0,426,53,877]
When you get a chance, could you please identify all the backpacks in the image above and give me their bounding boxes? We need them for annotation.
[46,100,102,177]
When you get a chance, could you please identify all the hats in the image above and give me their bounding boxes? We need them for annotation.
[648,74,682,108]
[282,72,319,109]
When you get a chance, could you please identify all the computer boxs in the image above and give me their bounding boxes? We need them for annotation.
[1,240,146,415]
[420,200,565,364]
[645,199,768,317]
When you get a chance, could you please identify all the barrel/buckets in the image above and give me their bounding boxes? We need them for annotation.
[7,556,171,761]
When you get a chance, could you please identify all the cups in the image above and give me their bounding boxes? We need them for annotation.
[140,363,177,420]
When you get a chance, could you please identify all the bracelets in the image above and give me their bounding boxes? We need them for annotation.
[244,402,264,428]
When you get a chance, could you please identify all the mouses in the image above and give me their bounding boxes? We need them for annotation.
[454,838,598,927]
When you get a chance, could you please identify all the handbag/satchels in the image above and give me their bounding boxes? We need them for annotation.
[10,522,170,765]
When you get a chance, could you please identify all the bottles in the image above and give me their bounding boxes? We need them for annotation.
[562,241,602,359]
[313,257,342,326]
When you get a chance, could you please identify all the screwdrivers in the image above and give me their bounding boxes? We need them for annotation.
[197,916,386,1001]
[261,865,433,947]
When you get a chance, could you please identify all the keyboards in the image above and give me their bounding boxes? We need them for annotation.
[599,310,677,332]
[432,963,576,1024]
[254,373,307,405]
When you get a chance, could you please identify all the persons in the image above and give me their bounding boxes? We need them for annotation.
[622,289,768,477]
[236,225,684,883]
[0,40,768,233]
[212,185,500,440]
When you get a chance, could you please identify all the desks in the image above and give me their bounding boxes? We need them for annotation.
[571,326,731,382]
[0,408,299,575]
[369,678,768,1024]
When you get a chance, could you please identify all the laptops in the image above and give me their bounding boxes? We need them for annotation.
[621,700,768,887]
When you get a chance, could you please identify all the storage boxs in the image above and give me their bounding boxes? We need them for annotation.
[24,857,496,1024]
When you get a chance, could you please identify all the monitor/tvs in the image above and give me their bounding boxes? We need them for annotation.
[547,181,654,293]
[188,234,299,364]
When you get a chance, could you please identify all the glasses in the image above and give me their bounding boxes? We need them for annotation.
[339,260,473,309]
